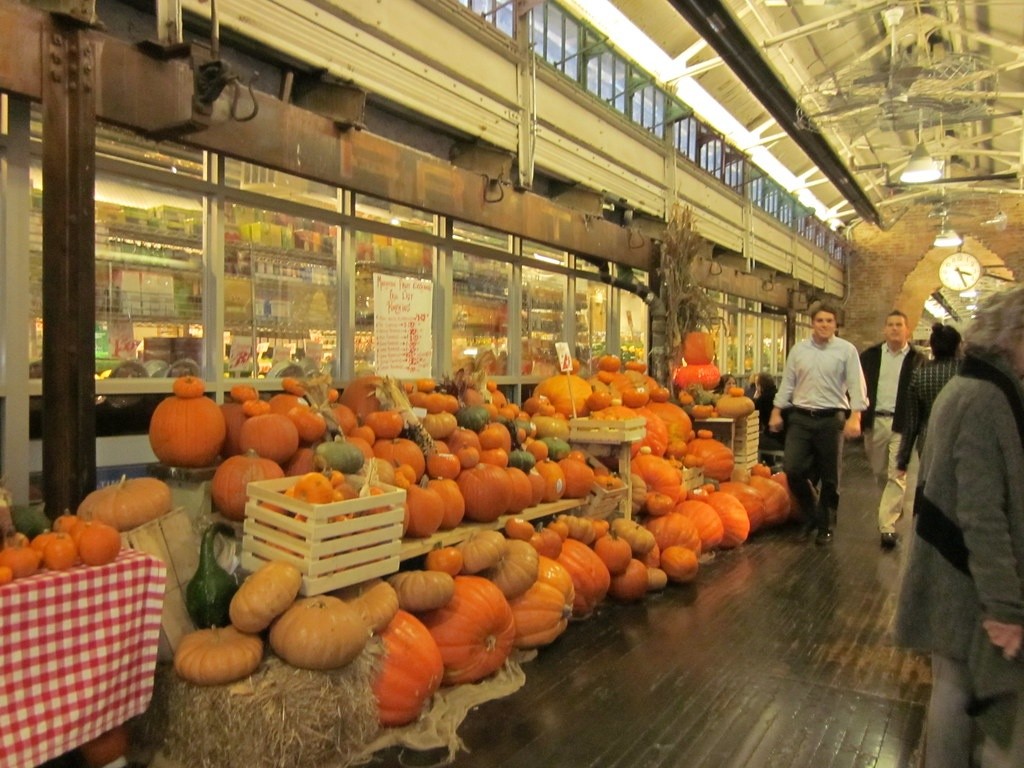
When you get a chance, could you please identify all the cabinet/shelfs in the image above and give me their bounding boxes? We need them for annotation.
[26,208,590,379]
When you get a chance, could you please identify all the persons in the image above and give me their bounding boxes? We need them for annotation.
[769,304,869,543]
[752,373,788,474]
[859,309,926,549]
[893,322,967,481]
[714,374,738,395]
[894,281,1024,768]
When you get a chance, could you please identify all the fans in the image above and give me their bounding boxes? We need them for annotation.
[812,7,968,120]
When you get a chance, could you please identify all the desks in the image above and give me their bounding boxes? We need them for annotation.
[0,548,167,768]
[147,457,590,562]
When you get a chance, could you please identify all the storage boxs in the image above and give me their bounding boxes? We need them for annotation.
[120,505,201,661]
[241,473,407,596]
[569,404,762,521]
[93,203,433,316]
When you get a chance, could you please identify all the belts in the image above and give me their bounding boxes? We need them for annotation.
[791,406,845,417]
[874,411,896,416]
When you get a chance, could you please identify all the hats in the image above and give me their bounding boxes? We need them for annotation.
[929,321,961,358]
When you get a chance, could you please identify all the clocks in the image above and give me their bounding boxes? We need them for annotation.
[938,251,981,291]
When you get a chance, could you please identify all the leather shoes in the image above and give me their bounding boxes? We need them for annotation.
[789,511,819,544]
[815,528,834,545]
[881,530,894,547]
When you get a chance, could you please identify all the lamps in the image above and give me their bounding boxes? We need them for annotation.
[901,107,940,183]
[934,186,962,246]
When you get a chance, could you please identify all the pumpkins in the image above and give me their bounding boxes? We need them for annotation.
[0,330,796,764]
